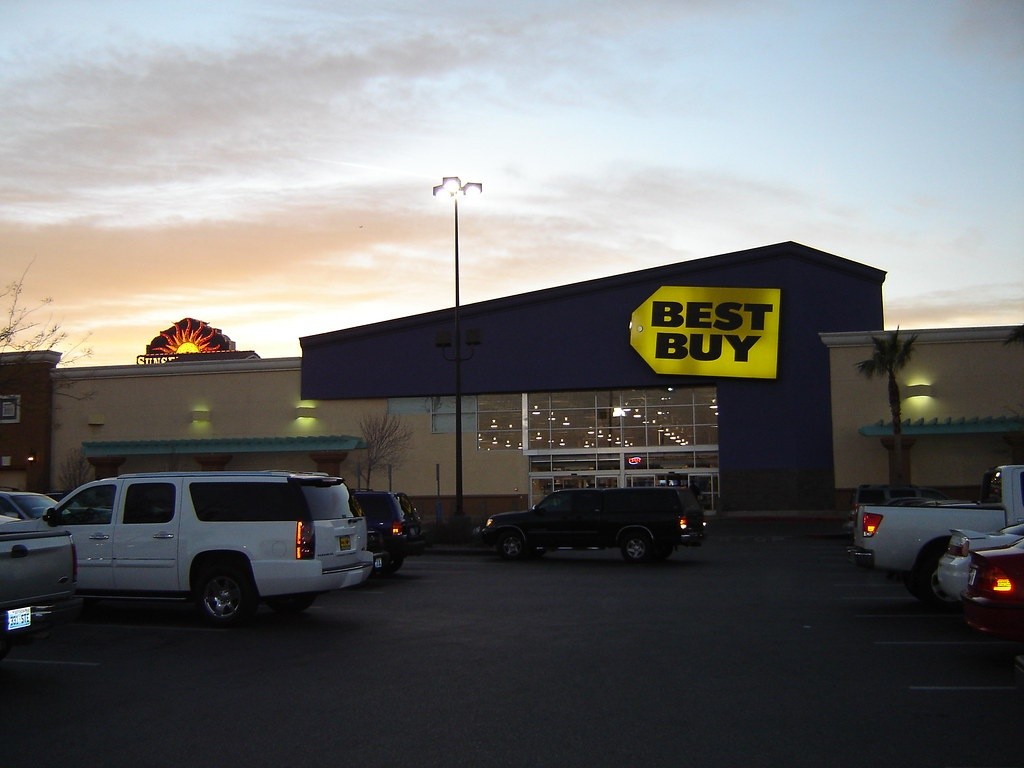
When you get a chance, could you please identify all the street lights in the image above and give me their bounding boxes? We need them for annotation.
[431,176,485,547]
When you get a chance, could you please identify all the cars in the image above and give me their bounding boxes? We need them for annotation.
[0,486,112,526]
[937,523,1024,647]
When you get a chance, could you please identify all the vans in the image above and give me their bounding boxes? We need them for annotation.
[856,482,951,508]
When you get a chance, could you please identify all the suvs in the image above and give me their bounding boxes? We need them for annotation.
[351,486,428,579]
[1,470,373,629]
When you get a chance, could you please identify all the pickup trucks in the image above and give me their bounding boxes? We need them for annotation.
[0,529,77,661]
[850,465,1024,613]
[479,485,707,566]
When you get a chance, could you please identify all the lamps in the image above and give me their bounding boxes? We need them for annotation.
[191,411,210,422]
[88,414,104,425]
[295,408,319,419]
[902,385,933,400]
